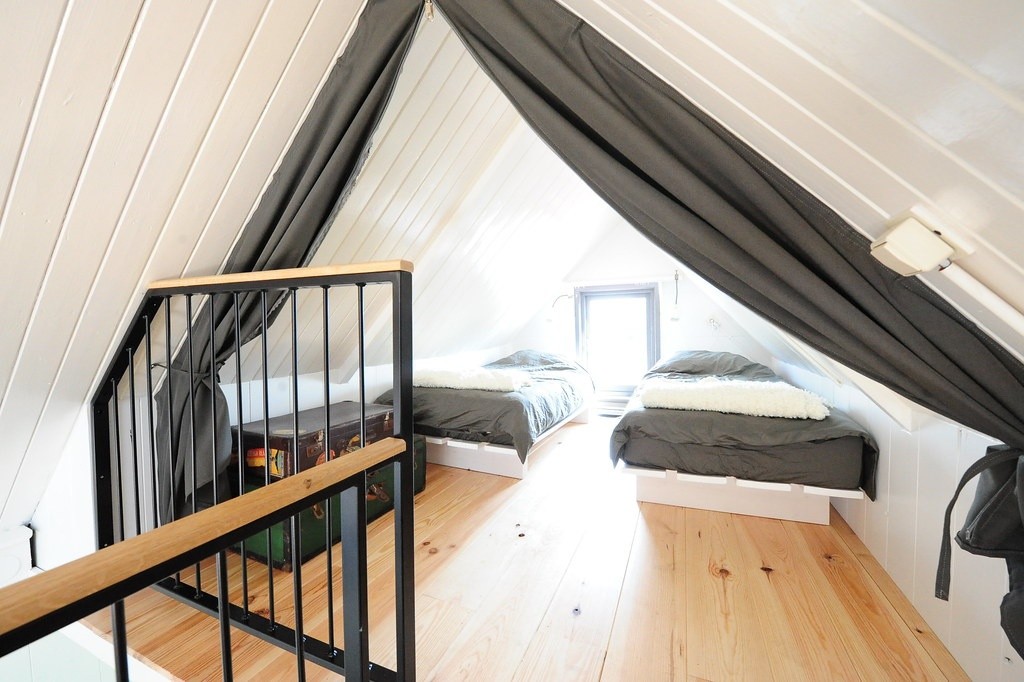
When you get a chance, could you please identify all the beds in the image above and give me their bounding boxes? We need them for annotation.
[374,349,596,480]
[610,349,880,526]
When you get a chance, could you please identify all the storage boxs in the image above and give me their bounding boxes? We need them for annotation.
[196,435,426,572]
[224,400,394,486]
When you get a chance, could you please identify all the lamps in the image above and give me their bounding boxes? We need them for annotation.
[667,271,686,320]
[870,220,949,277]
